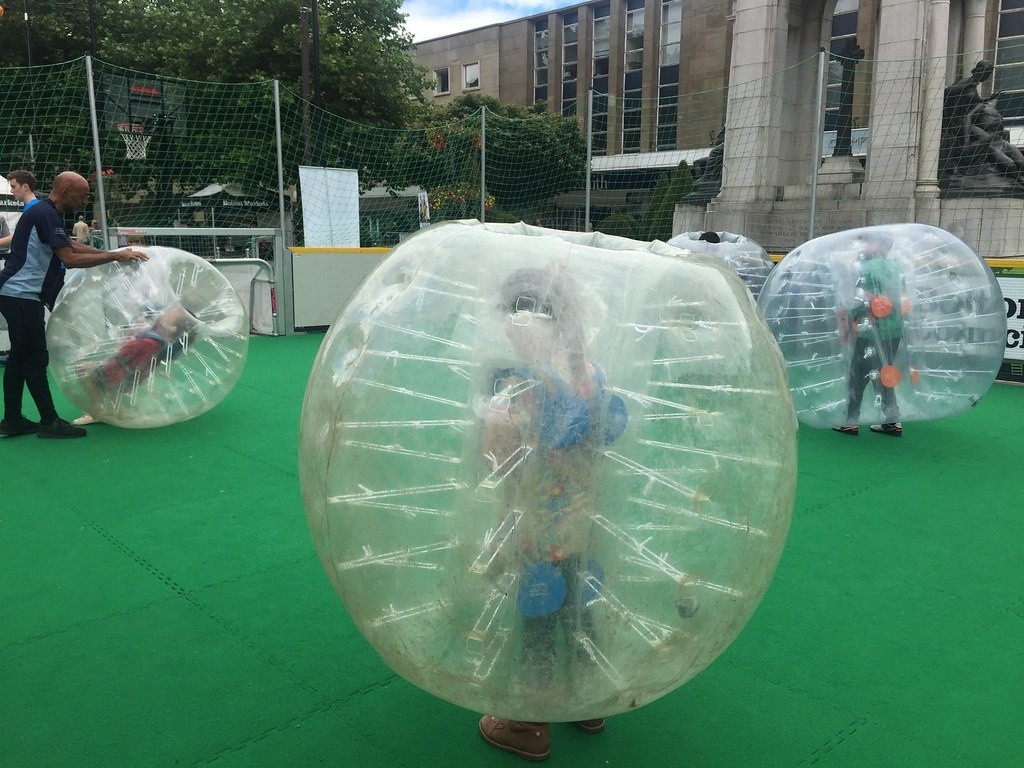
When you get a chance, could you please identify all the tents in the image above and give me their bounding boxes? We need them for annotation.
[176,182,294,261]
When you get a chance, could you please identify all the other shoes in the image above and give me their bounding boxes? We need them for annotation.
[832,424,859,435]
[36,416,88,438]
[572,717,606,733]
[72,413,102,425]
[0,415,40,437]
[479,713,552,761]
[869,422,902,436]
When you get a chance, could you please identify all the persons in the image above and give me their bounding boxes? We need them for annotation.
[0,170,41,252]
[698,231,722,245]
[960,98,1024,173]
[471,258,627,759]
[693,123,725,182]
[831,226,905,436]
[72,215,90,246]
[70,304,198,426]
[0,212,11,259]
[1,170,150,439]
[88,219,101,235]
[940,58,1000,178]
